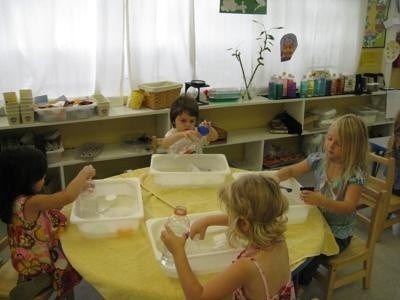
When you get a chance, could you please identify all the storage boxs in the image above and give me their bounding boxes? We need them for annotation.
[148,151,232,189]
[148,206,264,278]
[69,175,144,242]
[67,95,100,120]
[38,99,69,122]
[227,167,310,227]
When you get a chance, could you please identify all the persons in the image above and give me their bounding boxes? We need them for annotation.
[382,109,400,232]
[272,111,374,296]
[162,93,219,154]
[0,147,96,300]
[160,173,297,300]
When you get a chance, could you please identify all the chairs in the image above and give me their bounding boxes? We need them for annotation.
[307,188,388,300]
[356,153,398,242]
[2,236,73,299]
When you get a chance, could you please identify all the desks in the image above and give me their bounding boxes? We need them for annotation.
[59,163,339,299]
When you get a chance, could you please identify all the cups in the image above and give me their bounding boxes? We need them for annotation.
[300,186,316,191]
[129,90,145,110]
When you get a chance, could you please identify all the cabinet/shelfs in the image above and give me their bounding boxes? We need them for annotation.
[0,90,400,210]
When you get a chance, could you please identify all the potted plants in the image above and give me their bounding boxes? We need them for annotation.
[227,18,286,102]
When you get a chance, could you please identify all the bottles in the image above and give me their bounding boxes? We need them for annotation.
[159,205,190,271]
[166,124,209,161]
[268,70,385,99]
[77,173,98,220]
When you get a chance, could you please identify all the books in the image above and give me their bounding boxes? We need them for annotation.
[264,153,298,168]
[268,120,289,135]
